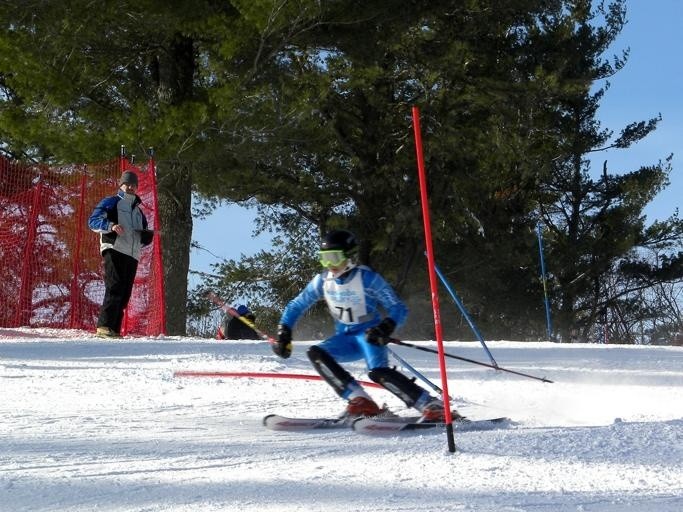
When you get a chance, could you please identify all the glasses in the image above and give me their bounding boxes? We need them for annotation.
[318,247,357,267]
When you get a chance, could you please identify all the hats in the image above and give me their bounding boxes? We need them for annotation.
[120,171,138,186]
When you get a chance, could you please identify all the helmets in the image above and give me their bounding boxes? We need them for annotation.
[319,230,359,269]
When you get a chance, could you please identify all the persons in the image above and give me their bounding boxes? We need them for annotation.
[228,305,250,339]
[88,171,153,341]
[272,230,462,425]
[240,314,259,340]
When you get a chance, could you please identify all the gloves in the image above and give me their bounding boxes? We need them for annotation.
[367,318,396,347]
[273,326,292,358]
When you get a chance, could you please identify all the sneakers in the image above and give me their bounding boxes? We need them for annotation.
[96,325,122,339]
[420,398,446,422]
[341,397,380,416]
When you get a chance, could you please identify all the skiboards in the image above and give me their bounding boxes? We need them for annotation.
[263,406,509,436]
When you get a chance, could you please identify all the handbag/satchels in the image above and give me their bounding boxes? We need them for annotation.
[228,306,258,339]
